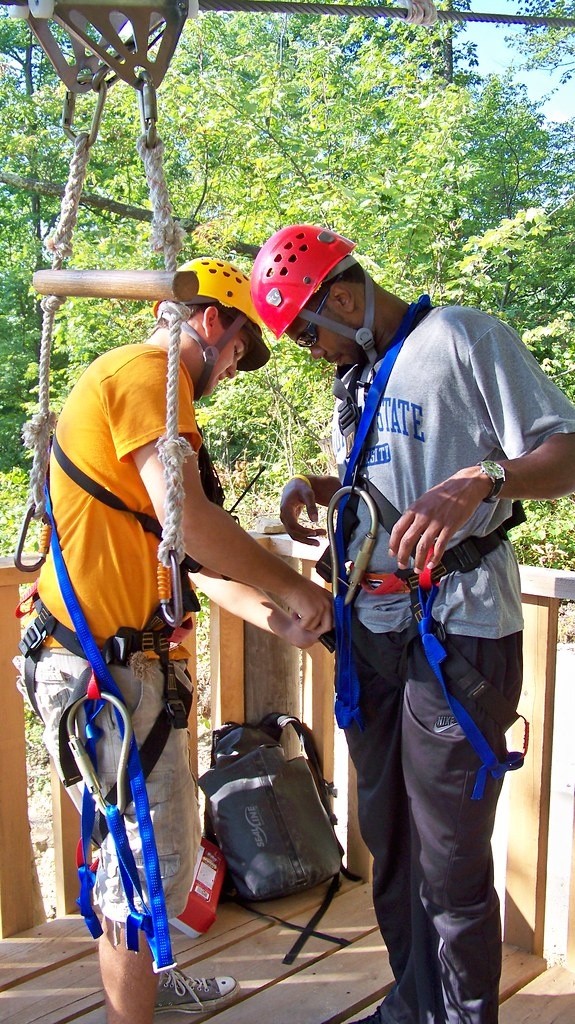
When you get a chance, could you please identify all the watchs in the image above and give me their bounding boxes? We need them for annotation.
[475,460,505,504]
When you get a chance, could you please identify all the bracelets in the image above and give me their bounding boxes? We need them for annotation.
[285,474,312,489]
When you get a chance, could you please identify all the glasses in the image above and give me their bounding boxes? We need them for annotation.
[295,275,342,347]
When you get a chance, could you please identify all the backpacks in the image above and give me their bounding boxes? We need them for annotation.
[199,711,363,965]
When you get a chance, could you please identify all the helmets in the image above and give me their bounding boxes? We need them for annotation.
[151,257,270,338]
[250,224,357,340]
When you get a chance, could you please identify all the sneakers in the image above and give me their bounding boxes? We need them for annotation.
[348,1006,381,1024]
[154,968,239,1015]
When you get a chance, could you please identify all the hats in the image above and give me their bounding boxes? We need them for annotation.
[227,308,270,371]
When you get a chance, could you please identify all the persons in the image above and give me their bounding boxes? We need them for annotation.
[249,224,575,1024]
[12,256,336,1024]
